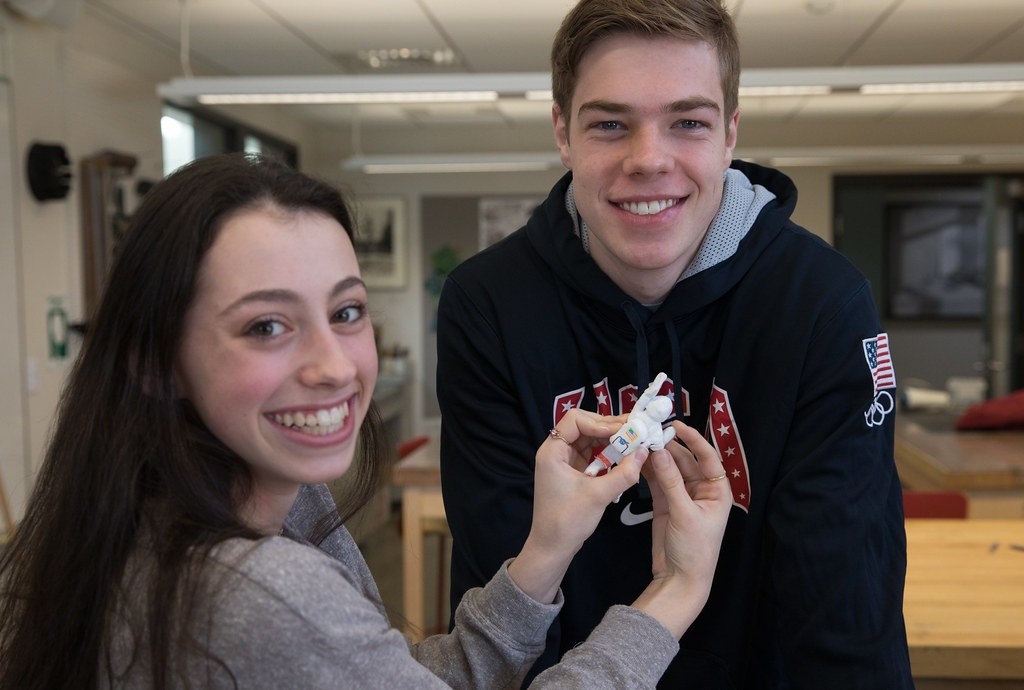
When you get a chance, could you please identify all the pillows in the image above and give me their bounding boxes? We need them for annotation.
[955,393,1024,431]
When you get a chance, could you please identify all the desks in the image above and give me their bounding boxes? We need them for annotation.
[888,409,1024,516]
[391,436,455,649]
[901,517,1024,689]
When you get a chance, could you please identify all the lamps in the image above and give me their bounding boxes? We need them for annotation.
[154,0,1024,177]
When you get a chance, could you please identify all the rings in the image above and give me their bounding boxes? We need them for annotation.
[705,470,726,482]
[551,428,570,446]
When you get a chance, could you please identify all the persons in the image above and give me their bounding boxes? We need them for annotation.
[0,151,734,690]
[436,1,915,690]
